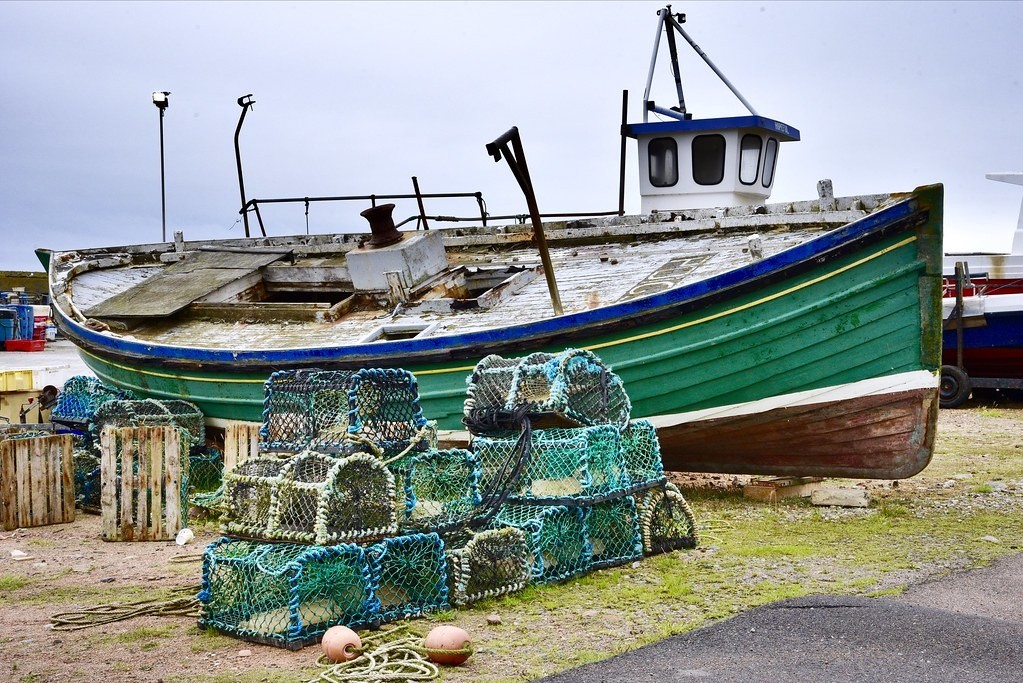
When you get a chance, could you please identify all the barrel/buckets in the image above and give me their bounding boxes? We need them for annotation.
[0,309,18,351]
[45,325,55,339]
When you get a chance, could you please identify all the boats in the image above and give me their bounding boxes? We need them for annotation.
[32,5,947,480]
[940,171,1023,409]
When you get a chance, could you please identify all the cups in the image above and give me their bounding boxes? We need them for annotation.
[175,528,194,547]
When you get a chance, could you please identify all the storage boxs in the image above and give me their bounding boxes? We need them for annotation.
[0,370,33,393]
[4,339,47,351]
